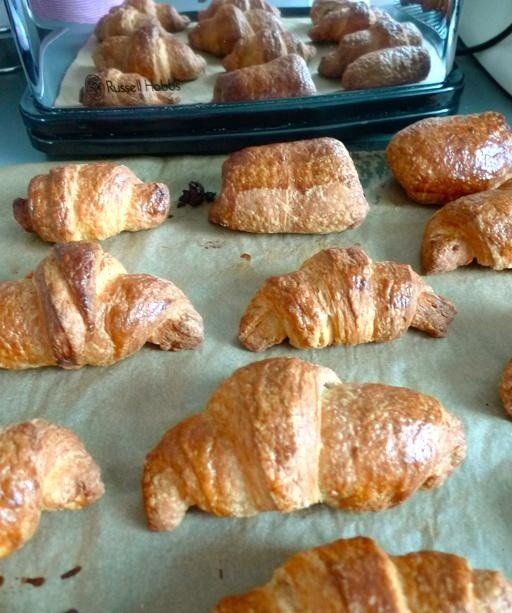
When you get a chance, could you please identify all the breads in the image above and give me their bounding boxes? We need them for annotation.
[142,356,467,533]
[419,180,512,275]
[343,46,431,89]
[318,20,424,78]
[91,24,206,89]
[307,2,391,43]
[109,0,191,33]
[188,3,286,59]
[307,0,350,23]
[80,68,182,107]
[221,26,318,73]
[208,137,370,235]
[12,162,172,246]
[496,358,512,422]
[94,8,172,41]
[238,246,458,353]
[209,53,318,100]
[0,238,203,370]
[386,110,512,206]
[195,0,282,23]
[211,534,512,613]
[0,418,106,561]
